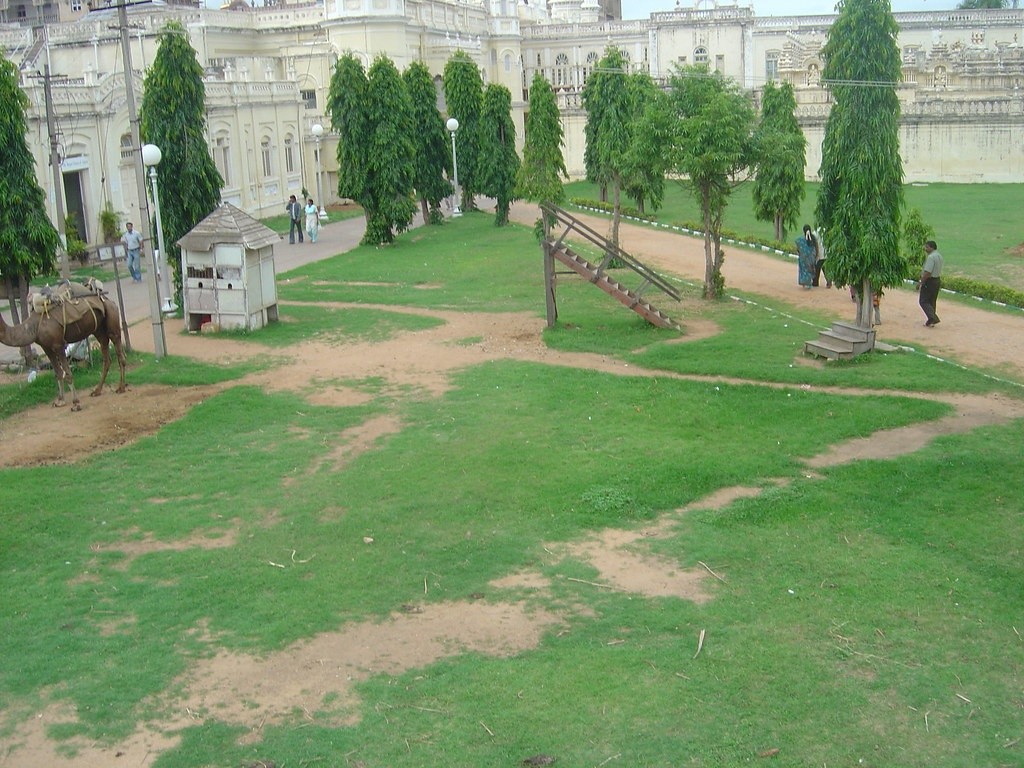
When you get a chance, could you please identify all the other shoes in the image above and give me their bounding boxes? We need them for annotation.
[826,280,832,288]
[926,318,941,326]
[812,283,818,286]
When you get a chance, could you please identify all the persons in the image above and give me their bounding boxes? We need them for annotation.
[286,195,303,244]
[850,283,881,325]
[808,65,819,86]
[916,241,943,328]
[305,199,319,243]
[445,176,455,211]
[123,222,144,284]
[934,67,947,86]
[812,224,832,288]
[795,224,819,290]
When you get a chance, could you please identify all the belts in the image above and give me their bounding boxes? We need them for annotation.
[928,276,939,279]
[130,248,139,251]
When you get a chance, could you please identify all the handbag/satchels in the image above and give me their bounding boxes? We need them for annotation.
[873,300,879,306]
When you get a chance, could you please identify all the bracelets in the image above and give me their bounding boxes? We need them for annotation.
[919,280,923,285]
[140,249,142,251]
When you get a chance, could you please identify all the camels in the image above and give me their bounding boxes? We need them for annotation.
[0,282,127,412]
